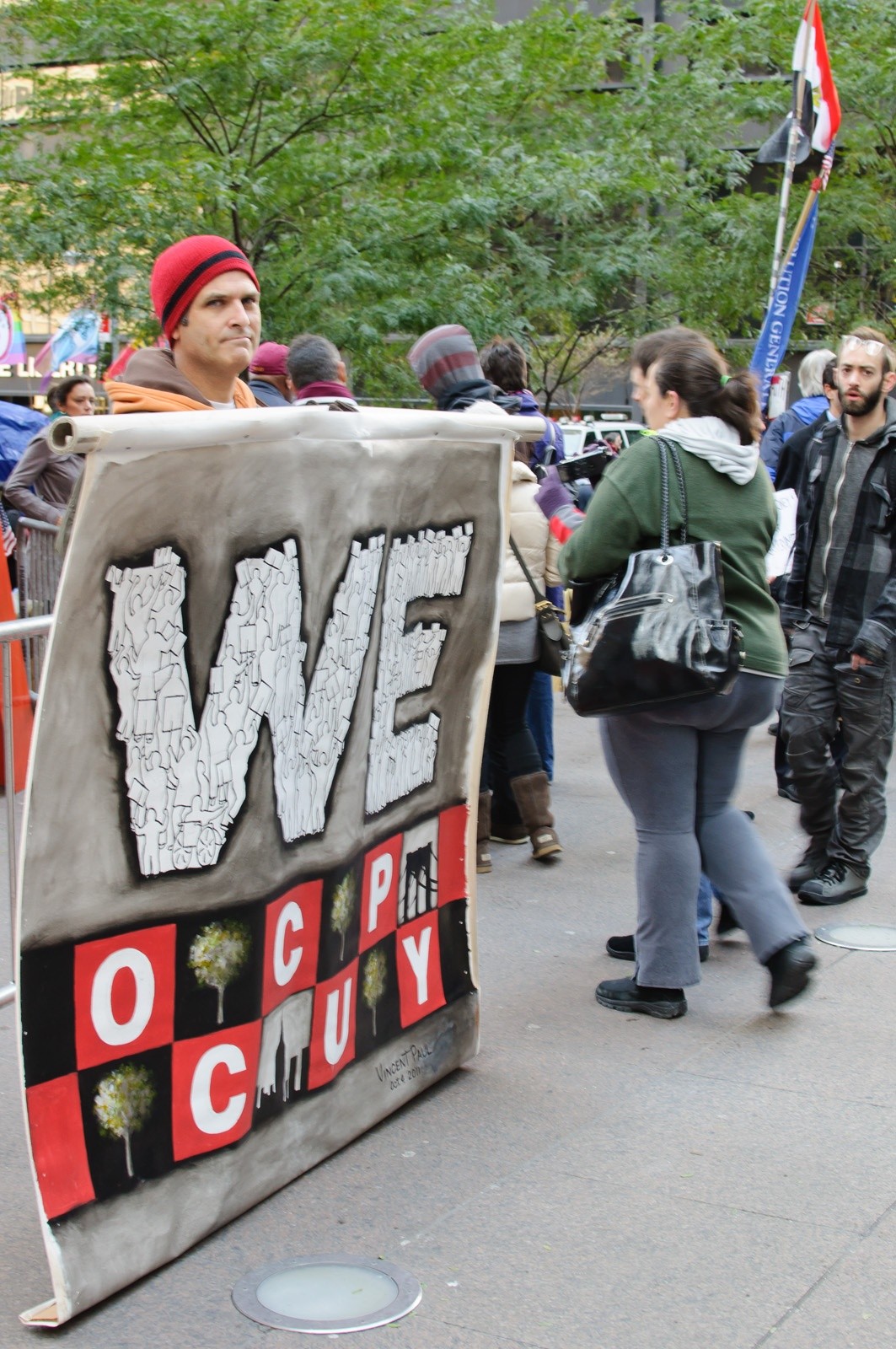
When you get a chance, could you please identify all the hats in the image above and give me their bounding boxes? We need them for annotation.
[151,234,260,351]
[248,342,291,376]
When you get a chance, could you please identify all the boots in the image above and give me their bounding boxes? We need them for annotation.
[509,770,563,860]
[476,790,493,874]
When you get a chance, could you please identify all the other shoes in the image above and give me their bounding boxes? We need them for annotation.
[767,723,779,737]
[778,784,802,804]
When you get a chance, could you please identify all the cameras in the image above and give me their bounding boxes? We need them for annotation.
[550,452,608,482]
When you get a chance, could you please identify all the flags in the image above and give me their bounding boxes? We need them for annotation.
[747,2,842,418]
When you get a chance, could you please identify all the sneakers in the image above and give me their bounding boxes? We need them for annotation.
[788,837,830,893]
[798,860,871,905]
[606,934,710,963]
[716,903,740,938]
[490,823,530,846]
[766,940,817,1008]
[595,976,688,1020]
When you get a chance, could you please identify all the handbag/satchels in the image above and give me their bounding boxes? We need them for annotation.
[535,597,570,677]
[562,434,747,720]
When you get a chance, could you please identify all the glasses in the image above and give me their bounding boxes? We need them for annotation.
[841,335,888,357]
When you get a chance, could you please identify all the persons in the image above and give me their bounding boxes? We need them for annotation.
[778,327,895,908]
[0,233,896,873]
[554,337,823,1021]
[531,331,759,962]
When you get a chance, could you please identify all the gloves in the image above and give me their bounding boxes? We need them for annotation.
[573,439,619,489]
[534,465,573,519]
